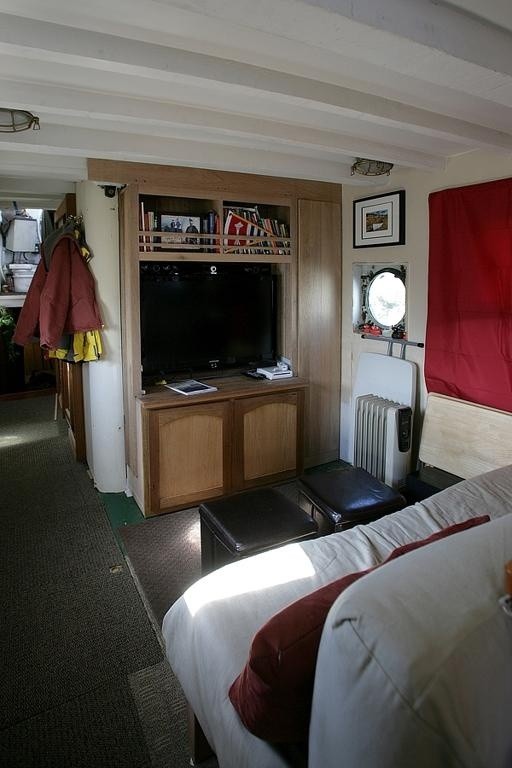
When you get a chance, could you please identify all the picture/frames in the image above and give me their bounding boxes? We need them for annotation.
[158,209,203,253]
[352,187,405,248]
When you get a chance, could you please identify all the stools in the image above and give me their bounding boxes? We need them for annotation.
[296,458,409,533]
[198,486,318,581]
[404,465,465,498]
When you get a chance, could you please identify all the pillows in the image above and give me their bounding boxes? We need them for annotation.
[222,515,491,744]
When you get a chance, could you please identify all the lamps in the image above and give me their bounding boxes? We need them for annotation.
[1,107,43,137]
[350,157,395,180]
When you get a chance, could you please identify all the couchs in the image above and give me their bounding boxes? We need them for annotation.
[160,461,512,766]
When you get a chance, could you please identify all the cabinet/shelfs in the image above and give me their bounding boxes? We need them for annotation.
[123,388,309,519]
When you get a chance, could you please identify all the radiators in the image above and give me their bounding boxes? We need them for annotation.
[352,394,412,491]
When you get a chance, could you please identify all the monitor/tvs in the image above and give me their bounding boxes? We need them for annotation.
[141,261,282,384]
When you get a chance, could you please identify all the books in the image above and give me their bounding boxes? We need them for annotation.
[139,202,290,255]
[164,379,217,396]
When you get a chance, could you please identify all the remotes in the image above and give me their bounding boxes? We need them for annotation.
[240,369,264,380]
